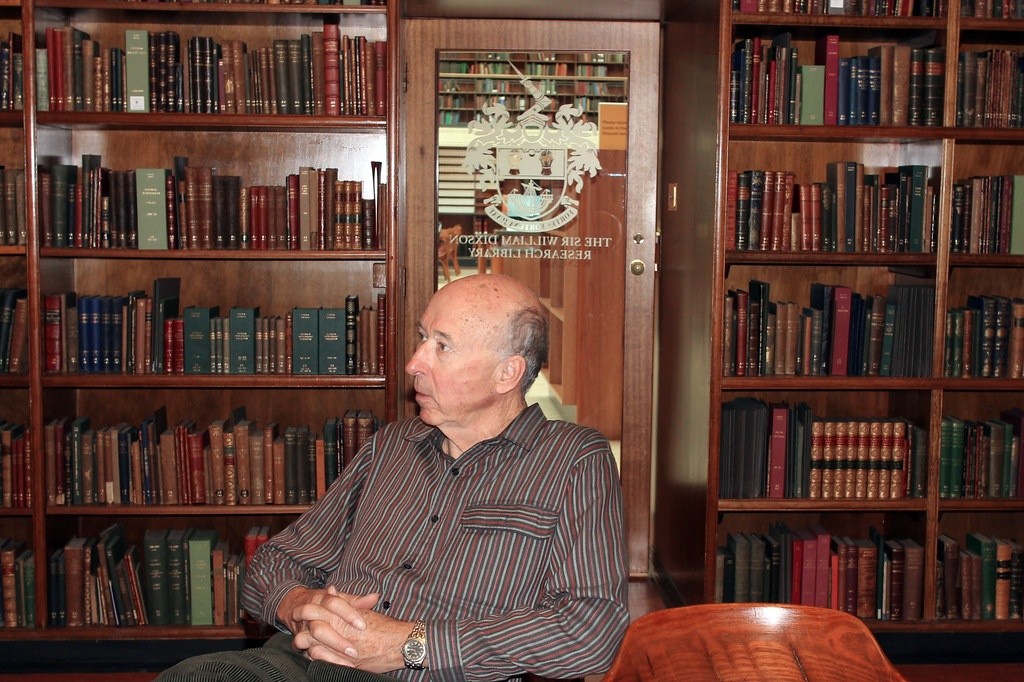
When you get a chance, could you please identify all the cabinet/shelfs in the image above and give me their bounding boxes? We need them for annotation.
[0,0,398,642]
[665,0,1024,682]
[398,0,664,580]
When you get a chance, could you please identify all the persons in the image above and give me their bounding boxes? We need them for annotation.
[152,274,630,681]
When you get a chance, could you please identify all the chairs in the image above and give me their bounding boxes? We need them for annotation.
[597,602,906,682]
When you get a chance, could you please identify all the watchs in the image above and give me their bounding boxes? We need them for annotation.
[400,619,429,670]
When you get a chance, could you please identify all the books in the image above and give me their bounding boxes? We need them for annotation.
[719,397,1024,501]
[715,522,1024,620]
[0,278,385,375]
[0,0,388,117]
[730,1,1024,132]
[0,405,384,511]
[722,280,1024,379]
[0,523,277,627]
[438,50,629,126]
[0,151,389,251]
[724,161,1024,256]
